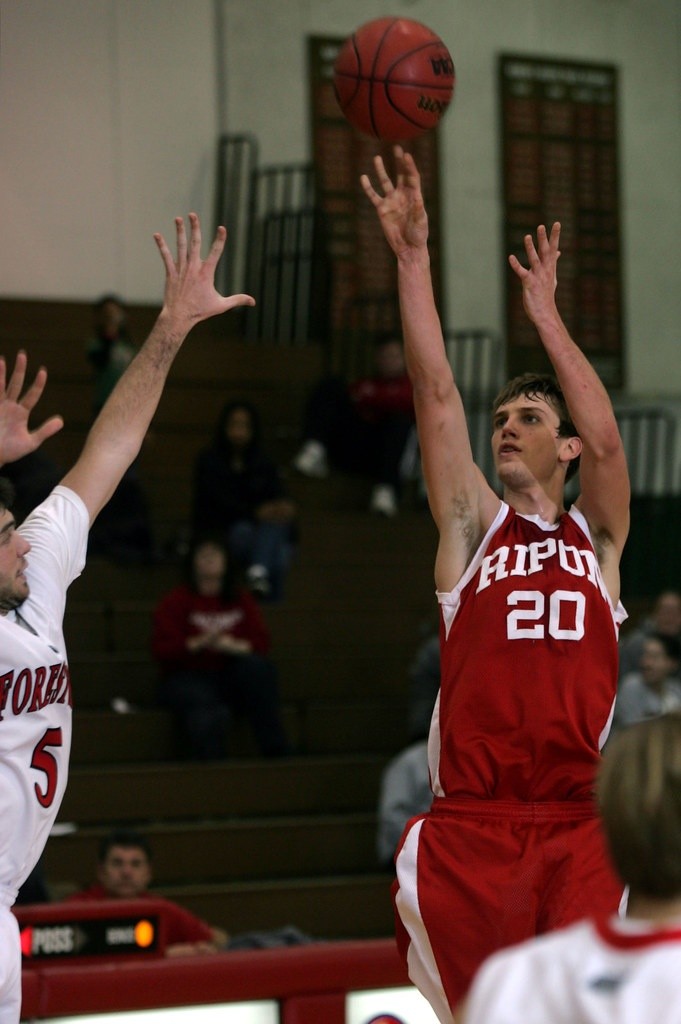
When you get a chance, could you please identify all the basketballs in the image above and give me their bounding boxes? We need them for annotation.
[334,15,455,143]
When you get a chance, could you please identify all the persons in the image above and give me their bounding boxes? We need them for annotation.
[76,293,681,862]
[361,145,630,1024]
[0,212,256,1024]
[465,712,681,1022]
[61,834,222,952]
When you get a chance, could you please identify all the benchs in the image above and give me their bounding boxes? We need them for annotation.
[0,293,681,954]
